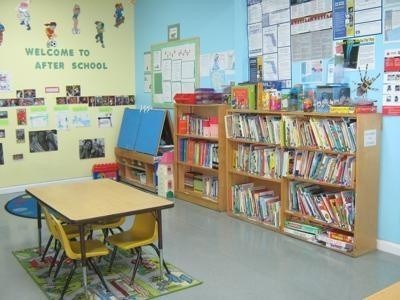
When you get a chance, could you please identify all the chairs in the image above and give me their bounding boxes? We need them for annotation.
[107,212,170,285]
[49,214,109,300]
[40,202,88,274]
[88,218,133,262]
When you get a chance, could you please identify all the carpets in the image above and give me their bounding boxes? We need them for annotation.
[5,193,46,219]
[12,232,203,300]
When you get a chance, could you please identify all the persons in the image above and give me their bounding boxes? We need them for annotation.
[30,131,58,151]
[80,139,105,159]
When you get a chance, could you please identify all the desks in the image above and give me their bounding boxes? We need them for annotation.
[364,282,400,300]
[25,177,174,300]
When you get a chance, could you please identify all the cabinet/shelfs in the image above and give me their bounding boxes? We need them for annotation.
[115,148,158,193]
[226,109,383,258]
[174,103,226,212]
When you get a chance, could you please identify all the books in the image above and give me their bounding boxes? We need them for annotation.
[177,112,357,253]
